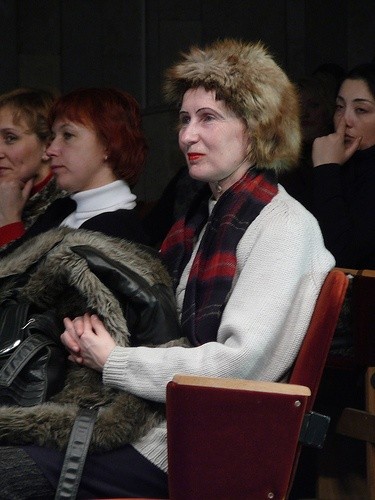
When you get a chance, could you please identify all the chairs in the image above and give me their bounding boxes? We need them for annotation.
[165,270,348,500]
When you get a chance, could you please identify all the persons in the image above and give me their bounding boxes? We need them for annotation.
[0,43,337,500]
[0,85,154,254]
[313,62,348,93]
[259,77,335,217]
[290,61,375,500]
[142,166,215,251]
[0,85,64,251]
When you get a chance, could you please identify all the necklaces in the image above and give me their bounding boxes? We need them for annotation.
[209,149,253,193]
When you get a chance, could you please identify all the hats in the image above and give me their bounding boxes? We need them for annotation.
[163,40,302,173]
